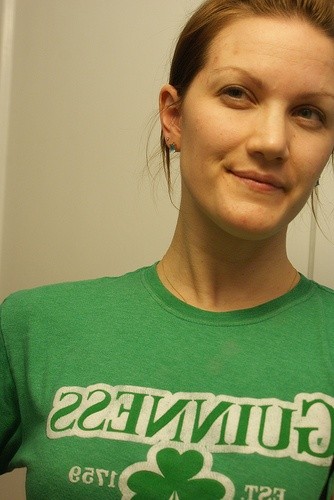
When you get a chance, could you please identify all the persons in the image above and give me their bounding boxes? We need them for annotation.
[0,0,333,500]
